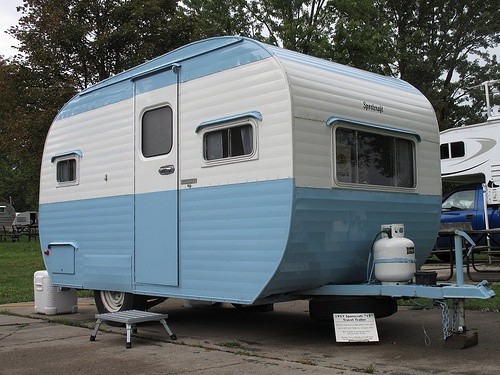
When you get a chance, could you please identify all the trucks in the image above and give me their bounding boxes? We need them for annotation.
[0,196,38,237]
[439,79,500,208]
[37,36,496,330]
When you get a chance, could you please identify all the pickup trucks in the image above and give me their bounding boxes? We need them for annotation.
[433,180,500,262]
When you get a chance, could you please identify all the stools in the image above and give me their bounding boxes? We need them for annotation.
[90,309,177,349]
